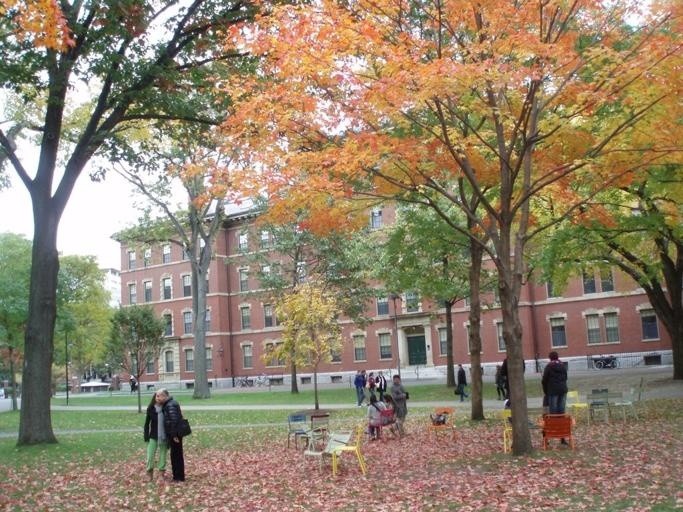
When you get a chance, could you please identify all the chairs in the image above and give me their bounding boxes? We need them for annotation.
[427,408,457,442]
[543,414,574,452]
[368,409,400,440]
[590,388,611,422]
[500,410,513,453]
[566,391,588,424]
[288,414,366,476]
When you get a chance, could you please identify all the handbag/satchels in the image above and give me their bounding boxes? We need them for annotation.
[172,419,192,438]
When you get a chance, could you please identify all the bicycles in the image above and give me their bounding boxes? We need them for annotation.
[233,375,249,388]
[254,372,270,387]
[593,354,621,370]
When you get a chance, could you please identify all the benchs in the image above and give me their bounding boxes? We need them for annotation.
[587,389,638,425]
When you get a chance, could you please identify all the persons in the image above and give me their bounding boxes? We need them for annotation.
[156,388,185,483]
[354,368,409,441]
[495,352,569,446]
[457,363,468,402]
[141,392,168,483]
[129,375,136,395]
